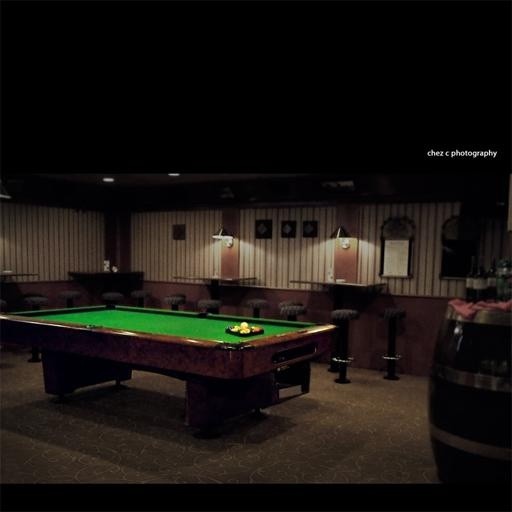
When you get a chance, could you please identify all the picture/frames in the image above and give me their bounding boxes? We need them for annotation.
[439,217,480,281]
[379,215,413,280]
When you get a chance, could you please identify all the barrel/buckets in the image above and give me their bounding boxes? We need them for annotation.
[427,299,511,485]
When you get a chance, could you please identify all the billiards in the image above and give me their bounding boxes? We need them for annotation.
[229,321,262,334]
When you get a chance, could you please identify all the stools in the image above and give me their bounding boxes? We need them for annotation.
[0,289,407,385]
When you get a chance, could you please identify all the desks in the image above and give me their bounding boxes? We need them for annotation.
[173,275,256,300]
[290,279,387,289]
[0,305,338,439]
[0,272,41,282]
[68,269,145,292]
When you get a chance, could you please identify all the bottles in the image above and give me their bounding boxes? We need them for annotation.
[463,254,511,302]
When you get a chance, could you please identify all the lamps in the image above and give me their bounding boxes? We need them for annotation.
[212,226,235,248]
[0,179,12,199]
[329,226,352,249]
